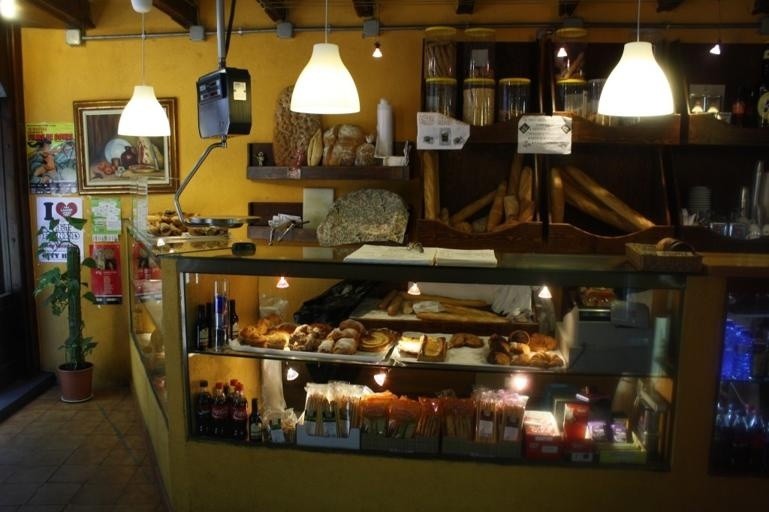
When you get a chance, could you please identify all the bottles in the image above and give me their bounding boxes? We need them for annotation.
[196,378,264,444]
[122,146,136,168]
[714,401,765,473]
[196,299,239,350]
[424,25,530,127]
[555,27,604,122]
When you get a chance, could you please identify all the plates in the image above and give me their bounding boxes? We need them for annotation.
[130,165,154,173]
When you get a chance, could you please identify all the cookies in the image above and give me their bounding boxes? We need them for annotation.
[302,382,524,444]
[423,42,610,126]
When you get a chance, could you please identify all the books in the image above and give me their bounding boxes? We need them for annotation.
[623,240,705,276]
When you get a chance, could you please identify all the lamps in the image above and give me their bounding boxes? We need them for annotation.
[287,1,361,117]
[116,2,173,139]
[597,0,676,119]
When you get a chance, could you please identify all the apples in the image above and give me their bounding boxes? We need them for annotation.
[98,162,115,175]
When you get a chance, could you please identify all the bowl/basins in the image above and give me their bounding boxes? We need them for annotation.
[184,217,229,236]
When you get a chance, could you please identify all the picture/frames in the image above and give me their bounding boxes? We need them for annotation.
[71,95,181,197]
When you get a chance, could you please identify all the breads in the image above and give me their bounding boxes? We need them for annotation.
[548,162,658,233]
[272,83,372,167]
[424,150,540,236]
[148,209,198,238]
[237,291,568,368]
[315,188,410,246]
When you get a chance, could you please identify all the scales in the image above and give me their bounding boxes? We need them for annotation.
[175,67,261,228]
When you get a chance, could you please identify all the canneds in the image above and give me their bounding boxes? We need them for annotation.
[710,221,746,239]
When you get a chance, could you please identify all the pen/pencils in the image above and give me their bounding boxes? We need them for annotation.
[269,222,295,246]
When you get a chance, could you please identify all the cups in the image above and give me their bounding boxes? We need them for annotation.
[687,186,712,219]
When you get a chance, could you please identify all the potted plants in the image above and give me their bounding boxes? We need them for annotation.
[31,214,103,408]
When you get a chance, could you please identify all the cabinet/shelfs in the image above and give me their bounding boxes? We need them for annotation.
[244,141,416,245]
